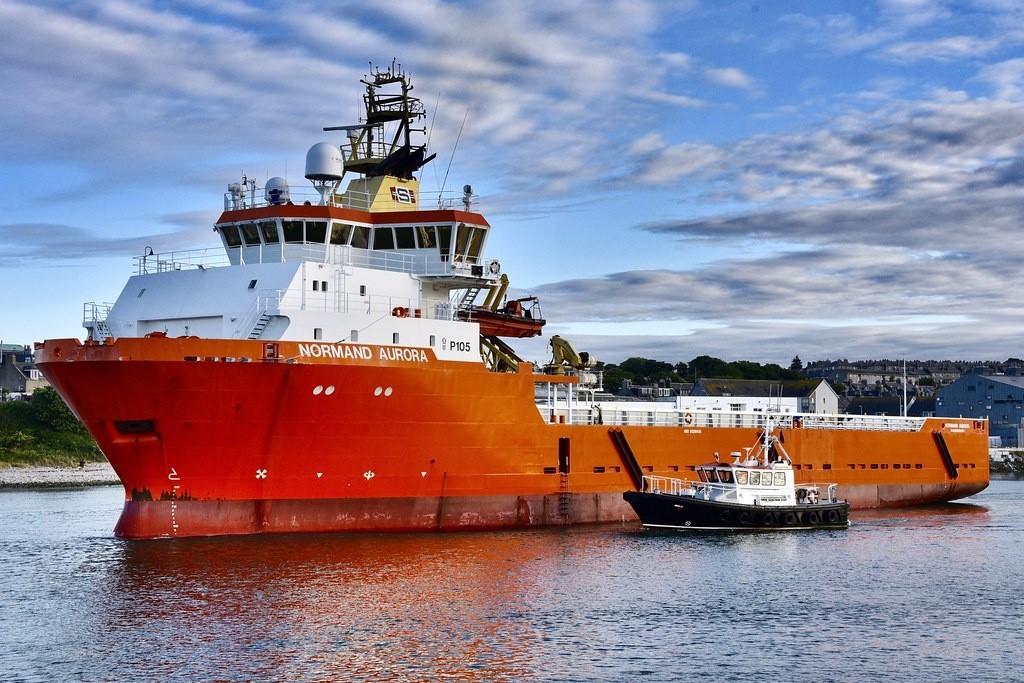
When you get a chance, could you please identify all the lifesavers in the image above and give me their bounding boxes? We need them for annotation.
[392,306,406,318]
[762,513,774,526]
[785,514,795,525]
[739,511,751,525]
[828,510,837,522]
[490,261,501,275]
[808,490,818,503]
[685,412,693,425]
[808,512,818,525]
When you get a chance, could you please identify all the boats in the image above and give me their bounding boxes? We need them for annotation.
[24,57,993,541]
[622,415,852,534]
